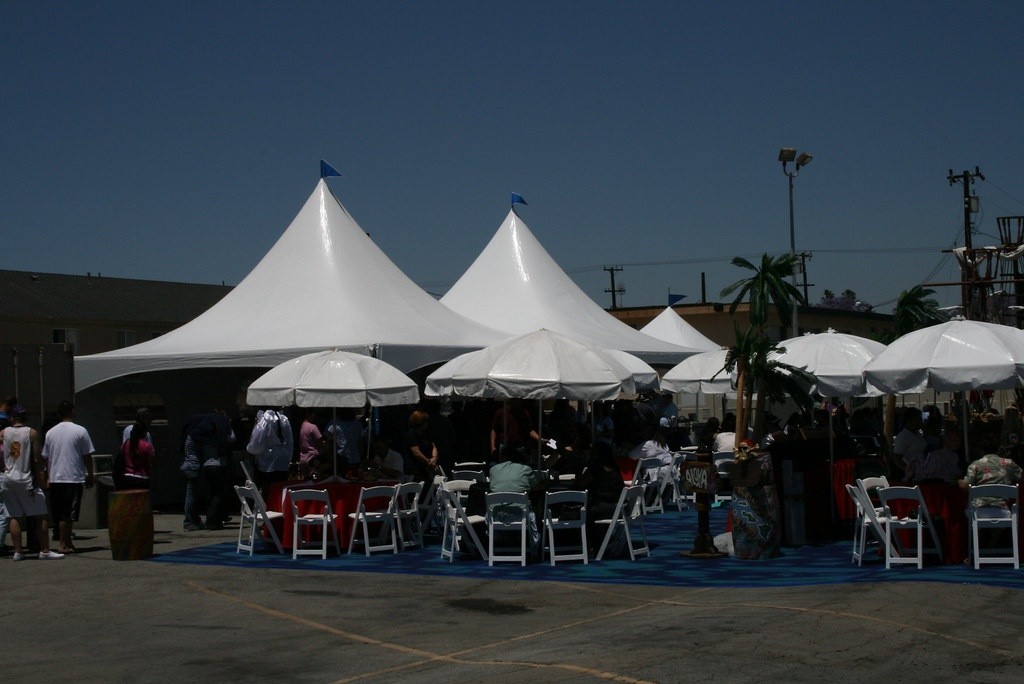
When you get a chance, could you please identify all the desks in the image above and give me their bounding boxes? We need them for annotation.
[262,476,401,550]
[965,481,1021,570]
[878,479,968,564]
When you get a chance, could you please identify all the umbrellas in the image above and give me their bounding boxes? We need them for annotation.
[660,348,791,417]
[767,328,887,480]
[425,328,659,473]
[246,349,419,481]
[864,314,1024,460]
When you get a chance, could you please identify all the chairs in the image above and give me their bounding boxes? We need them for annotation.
[843,474,941,570]
[229,447,734,567]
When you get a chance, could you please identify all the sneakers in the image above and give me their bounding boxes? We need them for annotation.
[39,549,65,559]
[13,553,27,560]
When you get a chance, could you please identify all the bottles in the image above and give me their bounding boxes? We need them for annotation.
[345,462,376,483]
[288,461,319,483]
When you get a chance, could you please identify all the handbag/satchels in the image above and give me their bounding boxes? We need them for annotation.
[111,439,128,483]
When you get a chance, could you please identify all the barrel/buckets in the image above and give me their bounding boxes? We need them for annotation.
[107,489,154,560]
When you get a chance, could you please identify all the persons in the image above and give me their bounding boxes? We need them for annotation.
[697,411,779,474]
[182,395,677,531]
[0,395,64,559]
[122,407,155,489]
[782,404,1024,566]
[42,400,94,553]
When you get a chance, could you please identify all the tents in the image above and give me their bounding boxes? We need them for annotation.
[435,192,706,362]
[72,159,519,424]
[639,306,723,419]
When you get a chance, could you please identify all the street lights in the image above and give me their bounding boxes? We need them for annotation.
[778,145,816,331]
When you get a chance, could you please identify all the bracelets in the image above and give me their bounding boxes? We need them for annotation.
[378,466,381,469]
[433,456,438,460]
